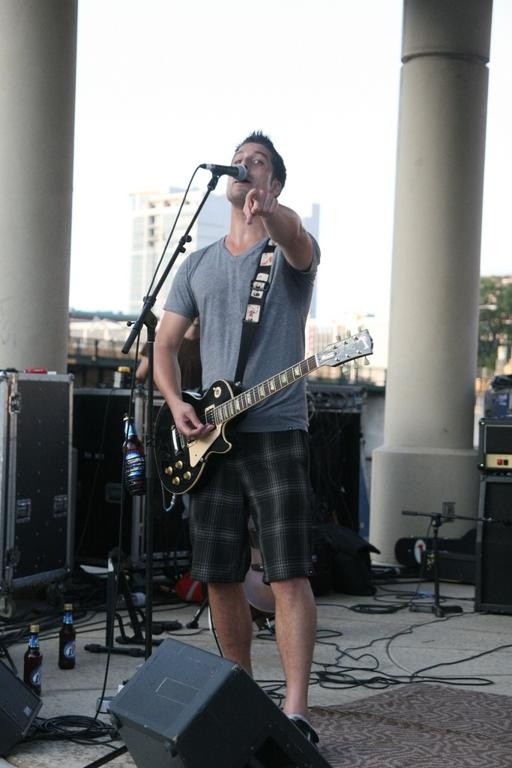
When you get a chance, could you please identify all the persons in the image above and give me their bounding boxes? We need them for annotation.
[133,309,206,606]
[147,123,325,756]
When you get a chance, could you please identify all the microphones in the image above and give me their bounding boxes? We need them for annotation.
[201,164,247,183]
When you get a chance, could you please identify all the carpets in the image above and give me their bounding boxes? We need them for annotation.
[309,683,512,768]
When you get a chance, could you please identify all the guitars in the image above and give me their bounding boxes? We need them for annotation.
[240,515,274,615]
[151,328,372,495]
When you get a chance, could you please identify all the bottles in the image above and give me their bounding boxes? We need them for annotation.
[121,412,148,499]
[58,602,77,670]
[24,623,42,697]
